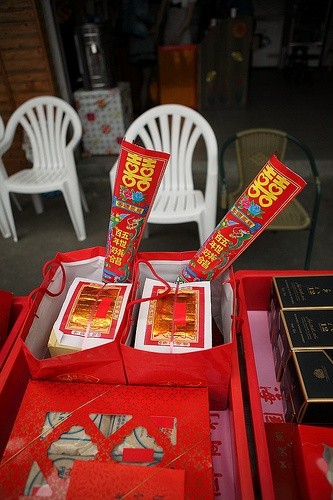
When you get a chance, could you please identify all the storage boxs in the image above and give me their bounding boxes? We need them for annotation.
[0,245,333,500]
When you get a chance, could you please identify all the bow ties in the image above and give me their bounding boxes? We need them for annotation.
[169,1,182,8]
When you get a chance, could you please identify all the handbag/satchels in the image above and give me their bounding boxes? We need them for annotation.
[19,245,128,385]
[118,251,244,410]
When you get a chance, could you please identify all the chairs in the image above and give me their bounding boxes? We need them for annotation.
[108,102,218,249]
[0,95,90,242]
[219,129,322,270]
[0,117,24,238]
